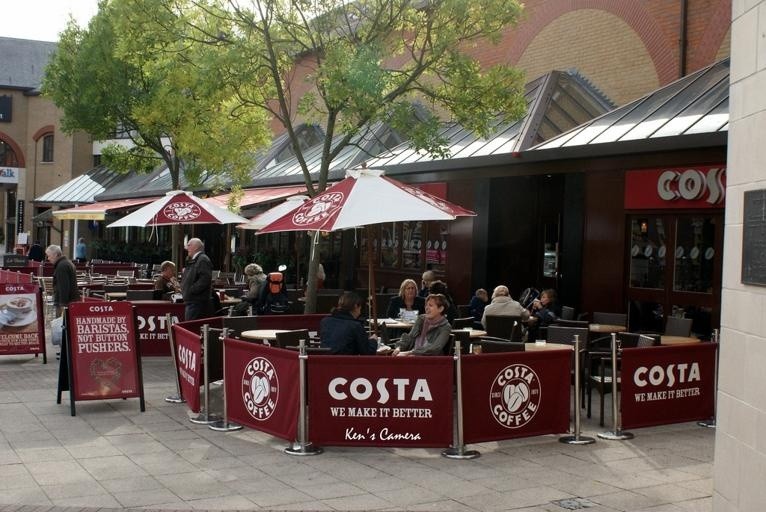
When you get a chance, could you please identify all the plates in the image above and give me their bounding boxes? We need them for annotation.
[0,309,37,327]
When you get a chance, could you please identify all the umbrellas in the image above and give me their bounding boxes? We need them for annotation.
[253,163,478,334]
[237,195,310,290]
[106,190,252,272]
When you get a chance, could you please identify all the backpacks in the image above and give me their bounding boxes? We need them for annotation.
[257,271,289,315]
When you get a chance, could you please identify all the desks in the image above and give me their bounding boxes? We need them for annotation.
[589,323,627,333]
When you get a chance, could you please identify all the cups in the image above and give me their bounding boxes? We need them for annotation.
[219,289,226,302]
[377,337,381,349]
[472,345,482,354]
[0,297,33,320]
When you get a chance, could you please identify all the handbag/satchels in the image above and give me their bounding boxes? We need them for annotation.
[50,311,64,347]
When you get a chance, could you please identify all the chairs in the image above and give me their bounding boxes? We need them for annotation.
[588,335,654,425]
[151,262,252,316]
[475,336,511,343]
[664,316,691,337]
[480,341,525,351]
[484,315,523,342]
[451,331,471,355]
[445,334,455,355]
[276,329,310,352]
[34,270,137,301]
[454,317,475,330]
[381,321,401,348]
[619,333,662,347]
[557,319,589,327]
[547,326,588,409]
[594,312,627,325]
[558,305,577,321]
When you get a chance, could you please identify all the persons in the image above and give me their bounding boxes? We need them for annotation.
[304,264,325,296]
[76,238,86,262]
[390,294,451,357]
[242,263,266,298]
[531,289,558,327]
[318,293,378,355]
[469,288,488,322]
[45,245,77,361]
[153,261,181,292]
[181,237,212,321]
[27,240,44,262]
[386,270,462,331]
[481,285,530,338]
